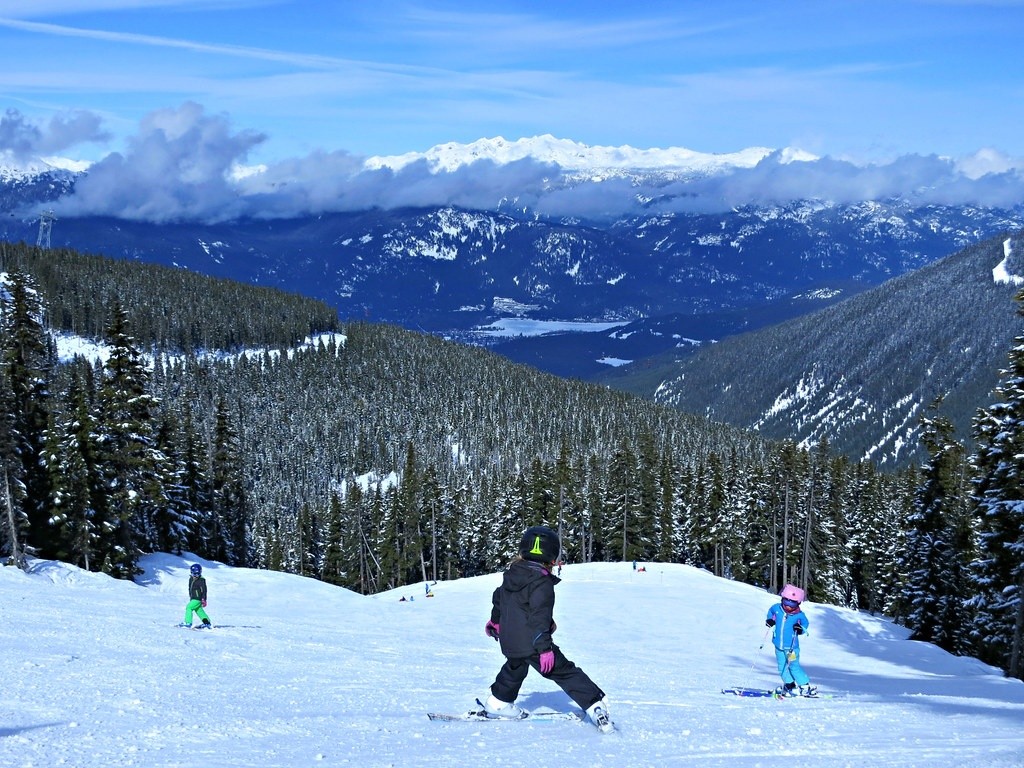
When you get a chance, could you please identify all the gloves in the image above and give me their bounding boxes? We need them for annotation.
[765,619,776,628]
[485,620,499,637]
[540,649,554,674]
[202,599,207,607]
[793,623,802,635]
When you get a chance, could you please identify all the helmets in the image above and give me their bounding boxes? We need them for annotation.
[518,526,562,563]
[191,564,202,578]
[781,584,805,604]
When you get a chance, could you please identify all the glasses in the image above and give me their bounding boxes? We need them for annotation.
[783,598,798,609]
[193,572,201,575]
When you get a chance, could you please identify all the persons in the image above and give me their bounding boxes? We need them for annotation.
[426,584,429,594]
[766,584,824,696]
[477,526,616,732]
[179,564,211,629]
[558,560,563,575]
[638,567,645,572]
[400,596,406,601]
[633,560,636,569]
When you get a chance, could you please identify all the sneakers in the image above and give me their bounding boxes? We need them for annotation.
[485,695,520,716]
[776,682,796,694]
[800,684,811,696]
[587,701,613,732]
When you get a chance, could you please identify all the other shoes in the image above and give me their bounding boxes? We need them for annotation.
[179,622,191,628]
[199,623,211,628]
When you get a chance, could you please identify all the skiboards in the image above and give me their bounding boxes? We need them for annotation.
[425,707,619,739]
[721,685,834,699]
